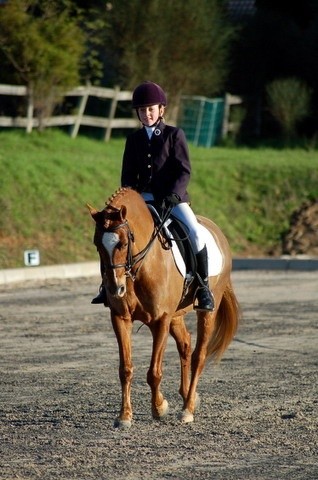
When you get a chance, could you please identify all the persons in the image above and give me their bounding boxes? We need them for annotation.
[90,81,215,310]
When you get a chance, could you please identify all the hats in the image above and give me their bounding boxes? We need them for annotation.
[132,82,166,110]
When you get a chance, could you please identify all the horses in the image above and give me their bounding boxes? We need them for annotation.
[86,187,240,428]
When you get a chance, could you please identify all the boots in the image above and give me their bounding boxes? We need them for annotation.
[193,244,215,310]
[91,259,108,304]
